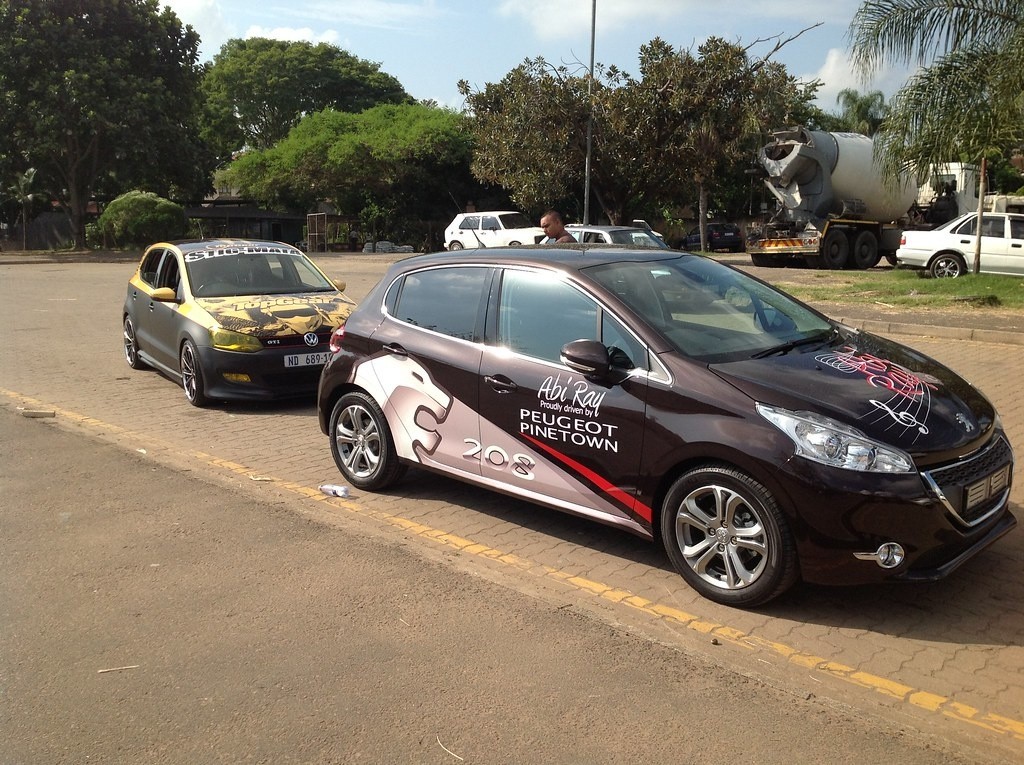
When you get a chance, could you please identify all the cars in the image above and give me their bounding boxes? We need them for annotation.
[120,238,356,414]
[564,224,592,228]
[317,250,1016,609]
[630,219,663,243]
[539,228,671,250]
[896,210,1024,285]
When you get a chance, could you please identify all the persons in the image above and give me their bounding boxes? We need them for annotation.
[350,229,357,248]
[540,210,577,243]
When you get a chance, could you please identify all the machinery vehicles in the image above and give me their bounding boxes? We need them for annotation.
[745,131,991,270]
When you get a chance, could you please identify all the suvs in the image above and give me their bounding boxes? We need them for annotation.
[443,211,547,250]
[682,223,745,252]
